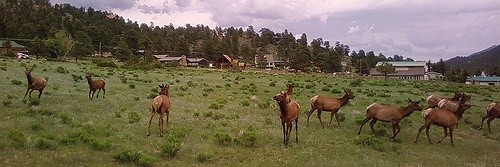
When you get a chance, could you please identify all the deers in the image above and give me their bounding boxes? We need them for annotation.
[414,91,471,146]
[479,103,500,134]
[272,91,301,146]
[277,83,295,105]
[357,98,423,142]
[306,89,355,127]
[85,75,107,100]
[146,83,170,137]
[23,66,47,100]
